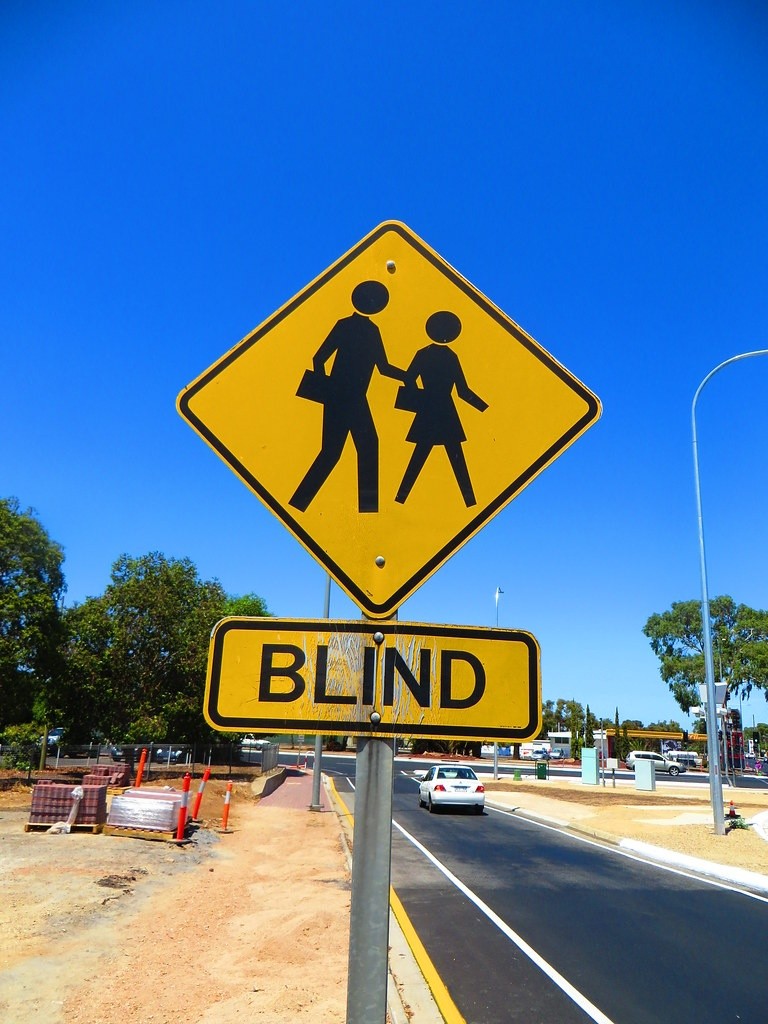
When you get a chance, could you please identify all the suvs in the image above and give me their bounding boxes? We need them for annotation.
[624,750,685,776]
[36,728,71,757]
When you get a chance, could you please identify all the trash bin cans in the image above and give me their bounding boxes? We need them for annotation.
[535,758,549,780]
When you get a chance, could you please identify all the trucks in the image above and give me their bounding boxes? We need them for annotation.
[550,746,570,760]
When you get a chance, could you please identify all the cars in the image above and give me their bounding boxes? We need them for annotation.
[532,751,549,760]
[156,746,190,764]
[748,752,756,758]
[419,765,485,813]
[110,741,147,762]
[242,732,270,750]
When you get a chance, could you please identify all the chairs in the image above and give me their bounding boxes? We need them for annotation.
[438,773,446,779]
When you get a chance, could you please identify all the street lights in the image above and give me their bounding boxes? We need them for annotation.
[495,586,504,628]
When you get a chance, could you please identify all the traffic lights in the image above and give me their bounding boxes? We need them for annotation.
[753,731,759,743]
